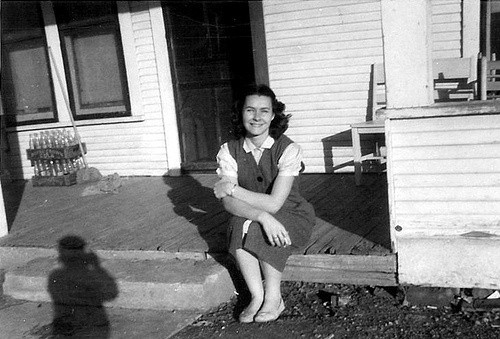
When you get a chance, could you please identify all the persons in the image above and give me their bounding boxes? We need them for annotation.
[214,84,315,322]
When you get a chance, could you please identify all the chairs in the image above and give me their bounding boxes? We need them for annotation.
[350,54,500,187]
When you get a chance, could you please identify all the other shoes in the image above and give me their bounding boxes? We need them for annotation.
[239,293,285,322]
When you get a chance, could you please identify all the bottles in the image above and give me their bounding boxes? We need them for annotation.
[29,129,86,176]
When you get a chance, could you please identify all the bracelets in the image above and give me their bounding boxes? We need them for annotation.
[231,184,237,197]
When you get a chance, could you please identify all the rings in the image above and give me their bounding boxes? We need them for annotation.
[274,235,279,240]
